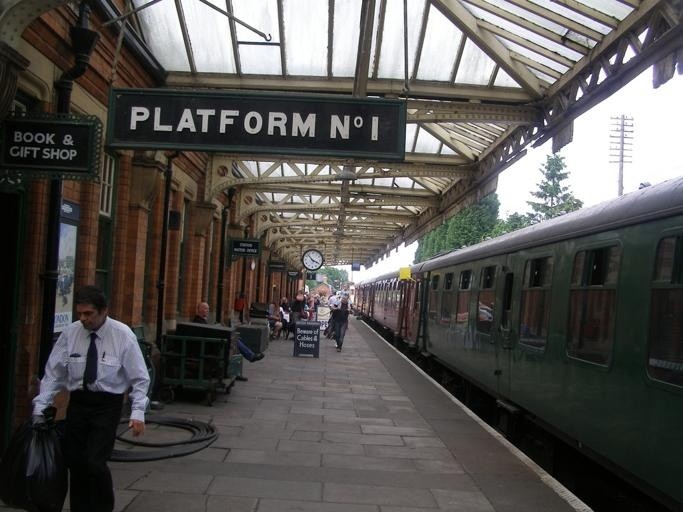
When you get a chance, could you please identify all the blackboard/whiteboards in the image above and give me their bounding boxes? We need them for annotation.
[294,321,320,354]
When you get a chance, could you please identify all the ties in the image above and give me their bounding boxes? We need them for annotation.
[84,333,99,386]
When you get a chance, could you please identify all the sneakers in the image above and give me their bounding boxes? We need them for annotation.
[235,375,249,382]
[249,352,265,363]
[336,345,342,352]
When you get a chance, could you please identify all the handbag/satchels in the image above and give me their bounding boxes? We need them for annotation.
[323,314,337,340]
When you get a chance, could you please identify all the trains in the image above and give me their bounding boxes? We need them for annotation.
[346,174,683,512]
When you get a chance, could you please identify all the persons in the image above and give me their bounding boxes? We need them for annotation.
[195,301,264,362]
[267,284,353,352]
[235,293,246,312]
[31,285,151,512]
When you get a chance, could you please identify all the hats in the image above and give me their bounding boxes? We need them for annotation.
[341,298,348,304]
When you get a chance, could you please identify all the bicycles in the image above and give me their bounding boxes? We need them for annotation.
[137,335,156,406]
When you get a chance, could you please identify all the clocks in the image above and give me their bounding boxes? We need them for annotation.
[301,249,323,271]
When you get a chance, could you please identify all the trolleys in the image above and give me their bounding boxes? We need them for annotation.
[158,332,239,404]
